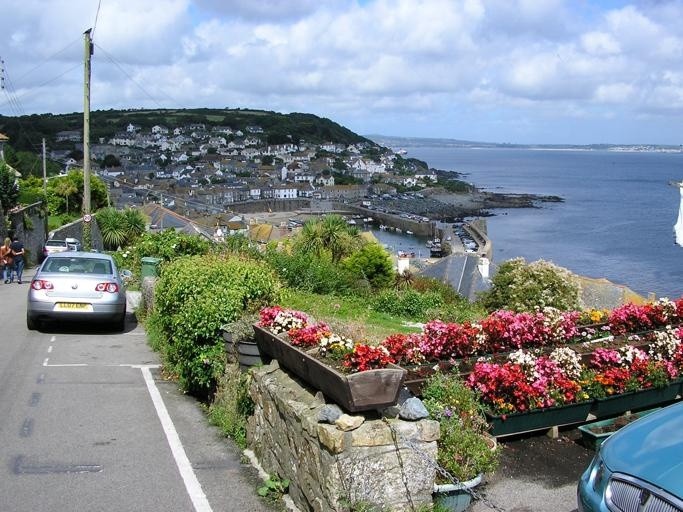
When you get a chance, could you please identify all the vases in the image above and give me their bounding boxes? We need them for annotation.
[427,475,484,511]
[220,322,236,364]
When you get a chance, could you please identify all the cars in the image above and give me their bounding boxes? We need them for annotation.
[66,238,81,251]
[362,190,430,223]
[286,218,305,231]
[451,224,477,251]
[23,249,134,336]
[568,397,682,512]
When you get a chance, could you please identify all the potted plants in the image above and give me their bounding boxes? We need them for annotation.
[231,315,263,375]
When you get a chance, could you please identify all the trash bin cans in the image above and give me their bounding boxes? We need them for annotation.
[140,256,164,290]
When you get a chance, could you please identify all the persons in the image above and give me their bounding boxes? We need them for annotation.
[2,237,25,284]
[0,238,22,284]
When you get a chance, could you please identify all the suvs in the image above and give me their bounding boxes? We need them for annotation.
[42,239,67,260]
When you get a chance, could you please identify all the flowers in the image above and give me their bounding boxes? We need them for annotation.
[423,371,497,482]
[389,298,679,414]
[259,307,389,373]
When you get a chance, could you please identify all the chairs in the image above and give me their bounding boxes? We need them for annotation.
[92,263,105,274]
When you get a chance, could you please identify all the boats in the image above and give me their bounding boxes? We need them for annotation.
[425,224,442,257]
[382,242,421,258]
[318,213,413,235]
